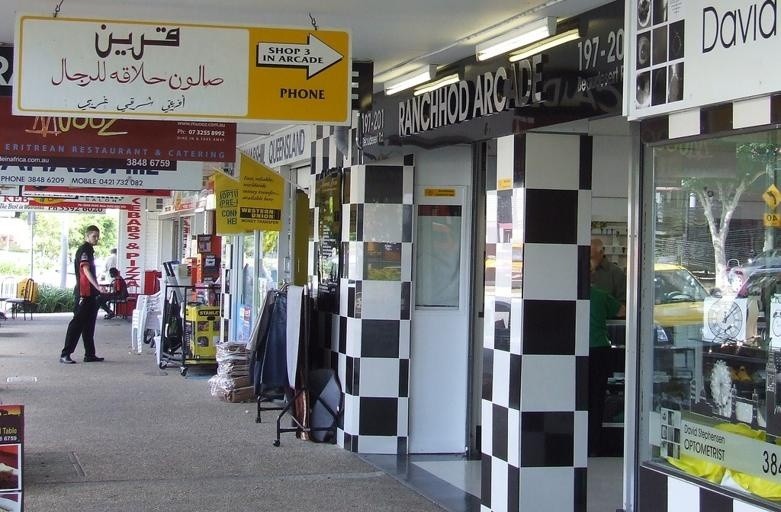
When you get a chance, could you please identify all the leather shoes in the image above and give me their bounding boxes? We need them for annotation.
[60,355,77,364]
[84,355,105,362]
[104,310,116,320]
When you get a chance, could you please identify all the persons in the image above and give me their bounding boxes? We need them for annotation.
[59,225,108,364]
[589,239,625,453]
[101,248,129,319]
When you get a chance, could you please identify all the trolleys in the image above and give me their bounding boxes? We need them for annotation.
[157,284,223,379]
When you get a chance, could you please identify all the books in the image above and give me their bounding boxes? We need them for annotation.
[0,405,24,512]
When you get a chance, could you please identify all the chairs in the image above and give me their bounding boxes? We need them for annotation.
[0,277,18,319]
[4,278,33,319]
[130,287,165,354]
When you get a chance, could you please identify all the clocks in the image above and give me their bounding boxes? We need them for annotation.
[708,299,742,340]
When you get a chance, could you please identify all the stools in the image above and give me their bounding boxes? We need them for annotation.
[107,296,137,321]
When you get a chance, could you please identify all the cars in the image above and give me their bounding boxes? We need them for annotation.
[699,266,780,402]
[652,261,710,324]
[242,259,314,309]
[728,247,780,295]
